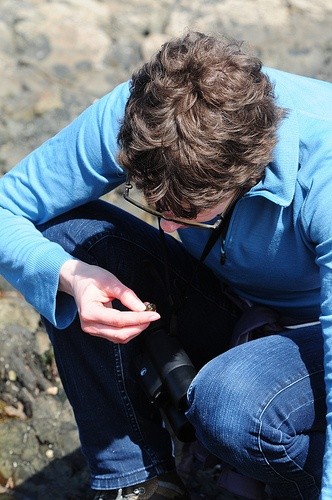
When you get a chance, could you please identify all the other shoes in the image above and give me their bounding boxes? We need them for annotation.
[93,471,190,500]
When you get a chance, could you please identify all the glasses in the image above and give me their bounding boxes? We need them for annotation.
[123,170,243,230]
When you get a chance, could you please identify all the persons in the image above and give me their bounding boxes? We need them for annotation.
[0,26,332,500]
